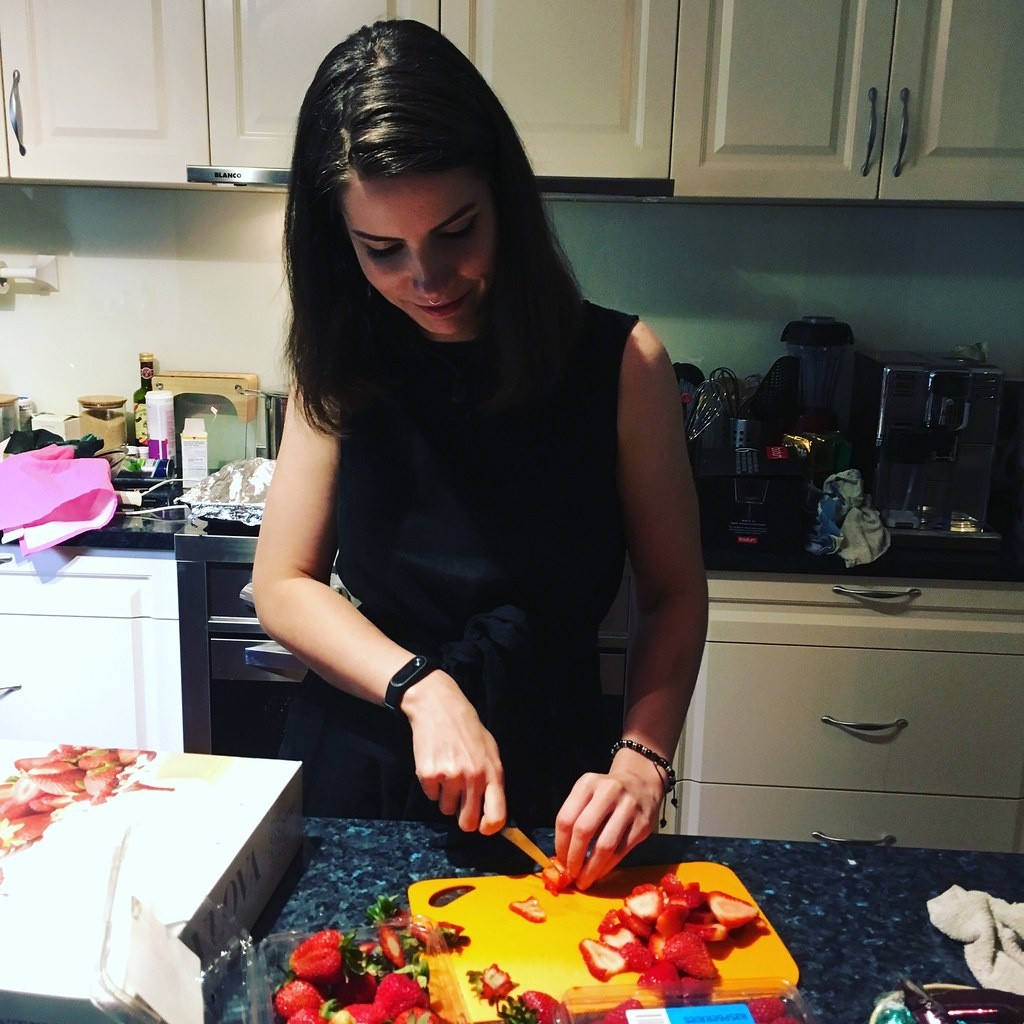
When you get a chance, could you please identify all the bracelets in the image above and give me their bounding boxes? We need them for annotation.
[607,740,678,828]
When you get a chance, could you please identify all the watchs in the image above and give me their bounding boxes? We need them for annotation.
[383,654,449,720]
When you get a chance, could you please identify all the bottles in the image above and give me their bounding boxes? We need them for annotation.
[132,351,154,447]
[17,397,33,432]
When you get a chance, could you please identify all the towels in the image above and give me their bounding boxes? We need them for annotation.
[926,884,1024,995]
[807,467,893,570]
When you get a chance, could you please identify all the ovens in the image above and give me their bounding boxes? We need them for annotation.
[207,634,447,824]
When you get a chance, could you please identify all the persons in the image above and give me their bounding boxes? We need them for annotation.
[252,18,709,890]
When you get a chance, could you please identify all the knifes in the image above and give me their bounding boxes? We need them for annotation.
[478,804,555,872]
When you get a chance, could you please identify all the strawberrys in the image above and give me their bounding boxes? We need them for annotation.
[0,745,149,889]
[269,854,804,1024]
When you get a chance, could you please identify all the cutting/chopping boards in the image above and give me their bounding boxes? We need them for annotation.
[408,862,799,1024]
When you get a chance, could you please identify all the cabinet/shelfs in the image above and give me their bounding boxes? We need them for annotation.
[674,571,1024,852]
[0,547,184,752]
[203,0,680,204]
[669,0,1024,212]
[0,0,214,192]
